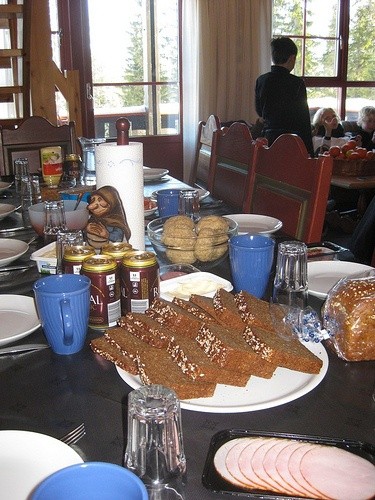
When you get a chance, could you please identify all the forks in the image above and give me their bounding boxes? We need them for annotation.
[60,422,86,446]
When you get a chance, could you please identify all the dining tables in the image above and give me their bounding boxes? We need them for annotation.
[0,165,375,500]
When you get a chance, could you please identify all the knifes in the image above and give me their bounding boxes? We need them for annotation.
[1,344,49,354]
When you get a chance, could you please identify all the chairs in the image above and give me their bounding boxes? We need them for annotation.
[188,114,332,243]
[0,116,76,179]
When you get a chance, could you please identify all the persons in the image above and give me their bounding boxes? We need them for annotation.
[315,108,344,155]
[85,186,131,248]
[255,37,313,157]
[351,106,375,150]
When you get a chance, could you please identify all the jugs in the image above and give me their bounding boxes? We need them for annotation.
[78,136,105,186]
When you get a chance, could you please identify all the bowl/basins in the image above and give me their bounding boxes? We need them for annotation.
[28,201,89,239]
[147,214,239,271]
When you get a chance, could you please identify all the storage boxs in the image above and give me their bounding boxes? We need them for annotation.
[333,158,375,177]
[30,241,57,274]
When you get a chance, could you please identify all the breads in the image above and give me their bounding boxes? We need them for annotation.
[322,277,375,362]
[88,289,323,401]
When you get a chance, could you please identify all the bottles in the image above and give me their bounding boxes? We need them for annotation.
[40,146,62,189]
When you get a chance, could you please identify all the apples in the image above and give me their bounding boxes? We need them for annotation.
[325,139,375,160]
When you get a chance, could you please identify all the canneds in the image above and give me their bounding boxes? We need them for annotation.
[65,154,82,185]
[61,242,160,330]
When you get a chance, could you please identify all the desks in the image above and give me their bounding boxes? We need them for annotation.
[330,172,375,236]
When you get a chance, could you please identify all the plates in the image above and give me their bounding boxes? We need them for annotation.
[160,262,234,304]
[143,168,210,216]
[305,241,375,300]
[114,339,329,413]
[201,428,375,500]
[223,213,284,236]
[0,181,41,346]
[1,429,84,500]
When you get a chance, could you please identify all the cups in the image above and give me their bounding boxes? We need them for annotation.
[178,191,200,216]
[14,158,91,354]
[228,234,309,298]
[31,383,185,500]
[156,189,179,216]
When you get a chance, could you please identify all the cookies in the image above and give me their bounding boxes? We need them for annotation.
[161,214,229,265]
